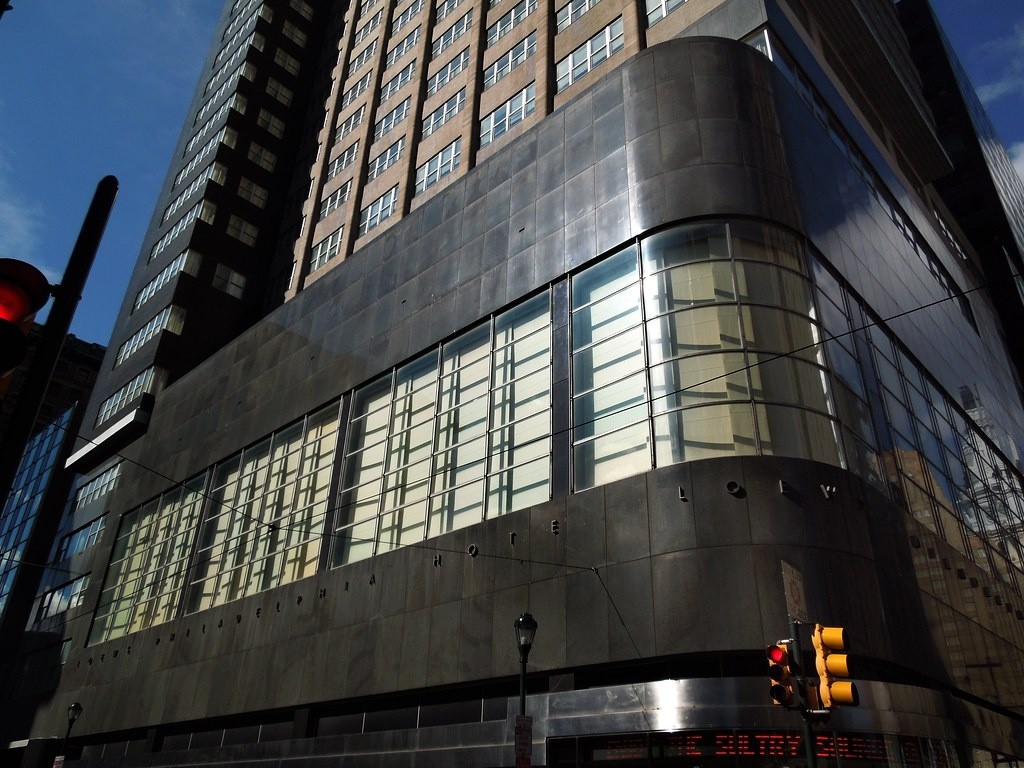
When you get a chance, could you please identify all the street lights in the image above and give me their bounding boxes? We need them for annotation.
[515,609,536,768]
[48,702,84,768]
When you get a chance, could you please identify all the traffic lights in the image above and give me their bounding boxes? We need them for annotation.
[1,257,49,378]
[765,639,795,710]
[812,621,860,708]
